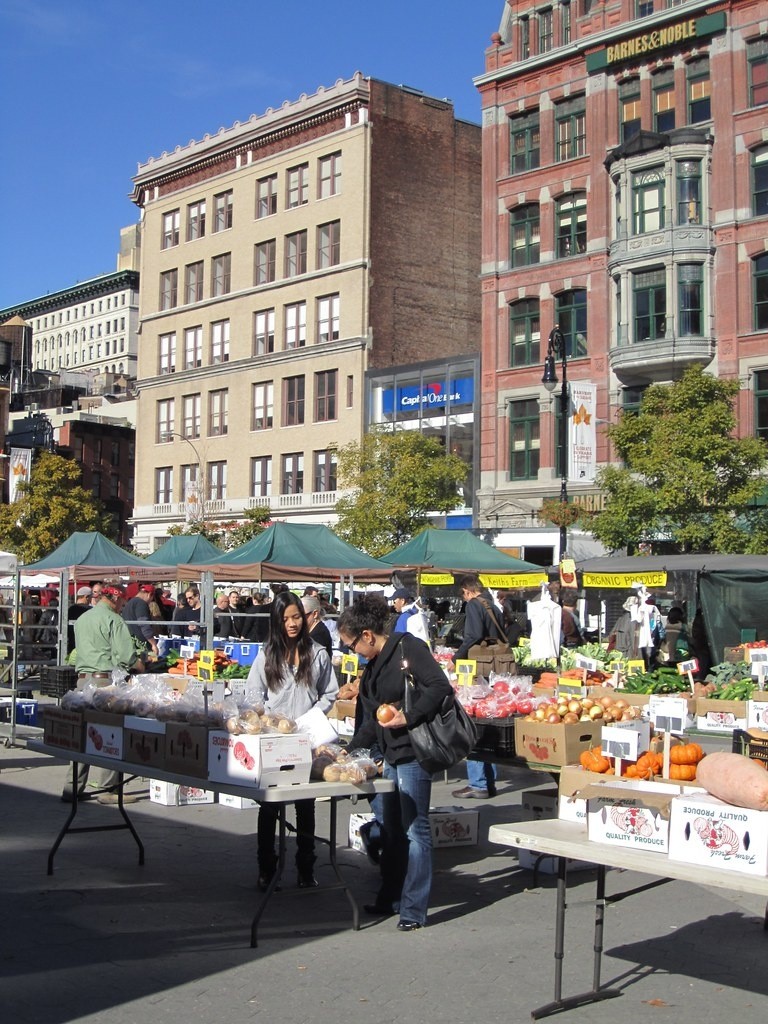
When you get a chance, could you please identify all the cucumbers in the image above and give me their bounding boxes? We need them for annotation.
[707,678,768,701]
[614,668,690,694]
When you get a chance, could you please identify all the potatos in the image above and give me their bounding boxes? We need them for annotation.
[61,685,298,734]
[311,744,378,784]
[680,678,737,699]
[338,676,360,702]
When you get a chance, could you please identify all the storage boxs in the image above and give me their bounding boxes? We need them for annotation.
[347,813,383,857]
[152,634,266,666]
[327,698,357,735]
[150,777,263,810]
[429,805,479,849]
[0,665,312,789]
[455,641,768,877]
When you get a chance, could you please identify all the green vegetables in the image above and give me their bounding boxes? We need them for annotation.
[703,660,752,683]
[509,640,630,671]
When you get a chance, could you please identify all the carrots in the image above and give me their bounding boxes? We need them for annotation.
[533,668,613,688]
[167,650,240,676]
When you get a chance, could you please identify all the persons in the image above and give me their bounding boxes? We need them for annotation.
[450,577,506,799]
[341,594,453,931]
[245,593,339,889]
[546,580,582,648]
[655,607,690,667]
[61,579,145,804]
[122,584,159,662]
[692,608,712,682]
[0,581,768,700]
[560,589,588,645]
[68,586,95,653]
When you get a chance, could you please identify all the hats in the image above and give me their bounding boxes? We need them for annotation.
[388,589,410,600]
[141,585,156,601]
[622,596,638,611]
[77,586,99,598]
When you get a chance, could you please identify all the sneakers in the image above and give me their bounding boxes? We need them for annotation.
[451,786,489,798]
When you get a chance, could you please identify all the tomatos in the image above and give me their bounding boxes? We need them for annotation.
[455,681,556,718]
[746,640,768,648]
[431,652,453,660]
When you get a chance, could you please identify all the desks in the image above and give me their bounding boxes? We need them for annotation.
[488,818,768,1017]
[466,751,563,888]
[25,739,395,949]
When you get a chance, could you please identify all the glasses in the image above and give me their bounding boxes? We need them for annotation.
[348,628,367,652]
[186,594,194,599]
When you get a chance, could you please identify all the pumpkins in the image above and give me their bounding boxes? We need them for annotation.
[695,752,768,811]
[579,740,767,780]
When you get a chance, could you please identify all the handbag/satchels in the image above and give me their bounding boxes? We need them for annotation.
[467,638,516,676]
[400,635,478,774]
[606,635,616,652]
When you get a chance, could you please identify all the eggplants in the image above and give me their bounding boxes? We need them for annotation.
[588,670,605,680]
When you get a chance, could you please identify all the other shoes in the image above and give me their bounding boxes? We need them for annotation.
[259,869,281,892]
[397,920,419,931]
[298,870,320,887]
[363,904,396,915]
[97,794,136,804]
[360,827,379,865]
[61,794,91,801]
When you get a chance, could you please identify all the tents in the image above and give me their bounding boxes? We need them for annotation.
[142,534,228,607]
[175,519,434,619]
[546,555,768,675]
[9,532,179,743]
[376,527,549,611]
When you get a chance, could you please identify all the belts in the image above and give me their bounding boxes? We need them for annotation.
[80,673,108,678]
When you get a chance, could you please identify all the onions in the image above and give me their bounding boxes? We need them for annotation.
[525,696,643,724]
[376,704,394,723]
